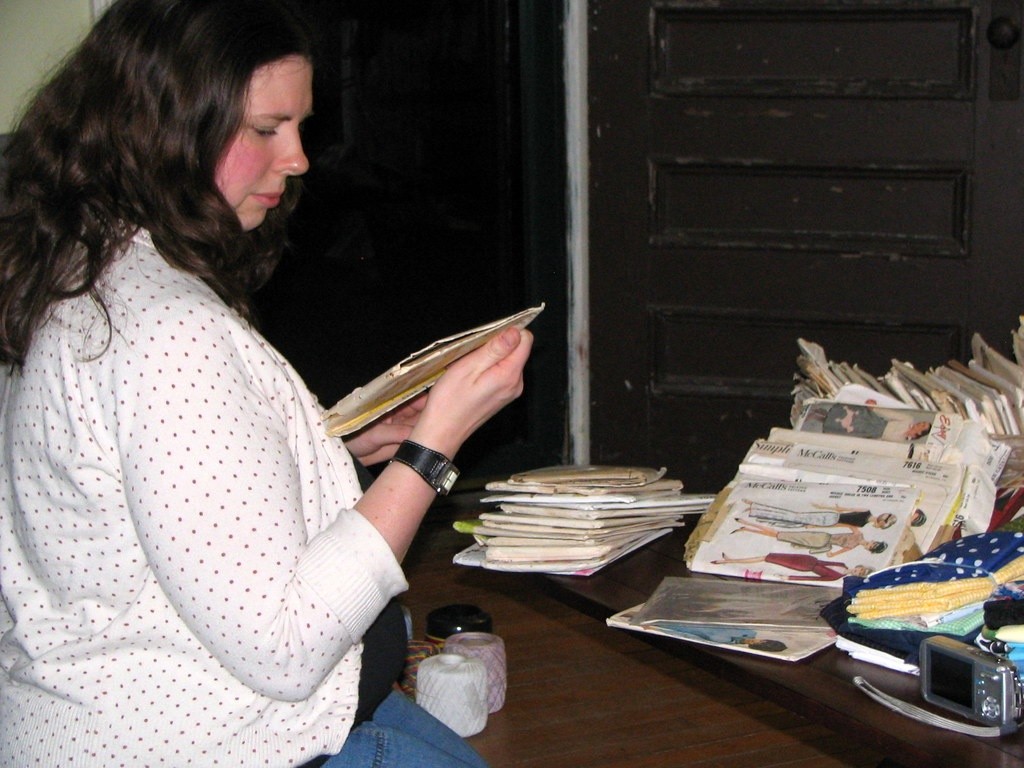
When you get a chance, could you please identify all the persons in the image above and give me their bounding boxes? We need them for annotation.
[1,0,532,768]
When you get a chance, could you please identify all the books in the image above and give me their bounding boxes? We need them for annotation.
[322,304,545,437]
[607,315,1023,663]
[452,466,717,576]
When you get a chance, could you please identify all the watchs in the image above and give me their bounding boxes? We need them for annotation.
[393,439,460,496]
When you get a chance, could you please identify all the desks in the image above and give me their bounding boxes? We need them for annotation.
[400,449,1024,768]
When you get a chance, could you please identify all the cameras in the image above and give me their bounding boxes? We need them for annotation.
[918,634,1024,727]
